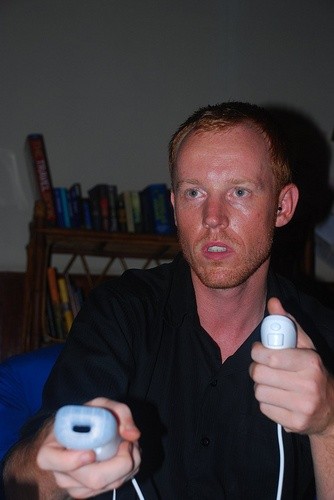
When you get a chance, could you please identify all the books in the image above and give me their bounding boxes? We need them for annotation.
[27,134,55,223]
[53,182,174,235]
[45,266,80,339]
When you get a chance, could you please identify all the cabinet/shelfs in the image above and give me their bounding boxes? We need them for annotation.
[15,196,316,348]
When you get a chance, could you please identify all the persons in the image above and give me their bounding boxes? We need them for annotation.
[0,102,333,500]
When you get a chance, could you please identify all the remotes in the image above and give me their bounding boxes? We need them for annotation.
[53,404,120,463]
